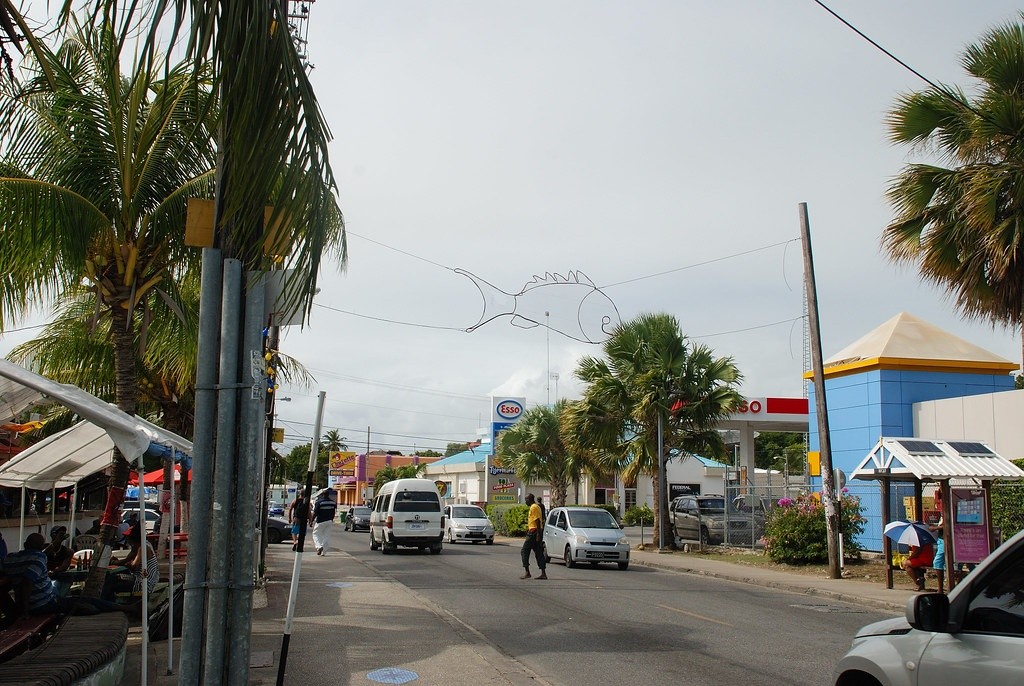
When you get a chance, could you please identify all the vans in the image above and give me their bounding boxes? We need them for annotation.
[367,478,446,555]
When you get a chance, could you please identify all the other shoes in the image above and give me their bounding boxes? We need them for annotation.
[293,543,297,551]
[317,547,325,556]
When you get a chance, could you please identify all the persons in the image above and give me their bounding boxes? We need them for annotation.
[902,509,946,593]
[519,493,547,579]
[0,513,160,613]
[289,489,336,555]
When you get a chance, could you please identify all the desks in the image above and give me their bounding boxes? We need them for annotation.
[51,561,128,601]
[145,533,188,558]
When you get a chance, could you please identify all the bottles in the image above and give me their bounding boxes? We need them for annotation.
[83,551,88,570]
[77,556,82,570]
[88,551,92,568]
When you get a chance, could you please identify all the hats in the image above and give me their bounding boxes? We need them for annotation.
[122,525,135,536]
[50,525,67,537]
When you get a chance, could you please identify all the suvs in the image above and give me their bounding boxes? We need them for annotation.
[732,493,784,527]
[669,494,760,547]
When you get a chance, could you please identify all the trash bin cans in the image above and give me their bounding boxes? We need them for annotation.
[340,511,347,523]
[368,504,372,510]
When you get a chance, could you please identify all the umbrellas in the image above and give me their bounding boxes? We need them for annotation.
[880,518,939,548]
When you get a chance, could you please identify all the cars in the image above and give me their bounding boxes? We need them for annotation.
[267,516,293,544]
[270,500,285,516]
[122,500,162,534]
[444,504,494,545]
[344,506,372,532]
[831,529,1023,686]
[541,507,630,570]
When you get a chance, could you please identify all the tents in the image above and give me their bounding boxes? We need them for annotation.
[0,358,194,686]
[132,463,194,486]
[0,403,194,571]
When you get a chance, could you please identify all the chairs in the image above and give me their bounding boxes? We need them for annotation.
[70,550,94,588]
[74,535,99,558]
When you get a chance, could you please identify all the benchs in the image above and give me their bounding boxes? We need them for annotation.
[0,570,55,663]
[105,570,186,638]
